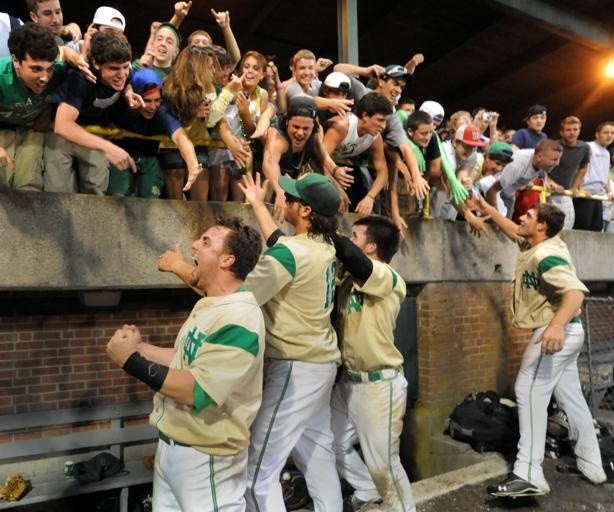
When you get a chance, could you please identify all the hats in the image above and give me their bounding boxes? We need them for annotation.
[325,70,352,90]
[419,100,446,125]
[277,173,340,217]
[93,5,127,32]
[288,96,317,119]
[131,68,163,98]
[484,140,514,157]
[455,124,488,147]
[384,65,413,79]
[522,104,548,124]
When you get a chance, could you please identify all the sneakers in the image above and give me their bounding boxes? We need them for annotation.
[553,460,591,477]
[486,472,548,499]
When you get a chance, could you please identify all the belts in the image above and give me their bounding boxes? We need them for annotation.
[344,368,400,384]
[158,429,194,448]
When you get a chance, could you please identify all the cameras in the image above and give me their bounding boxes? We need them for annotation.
[482,111,494,123]
[372,70,391,81]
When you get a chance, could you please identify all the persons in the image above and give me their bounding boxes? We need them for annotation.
[285,172,416,512]
[0,0,613,236]
[158,171,345,512]
[105,216,266,512]
[468,191,607,496]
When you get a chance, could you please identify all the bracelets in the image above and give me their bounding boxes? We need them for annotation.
[122,352,169,392]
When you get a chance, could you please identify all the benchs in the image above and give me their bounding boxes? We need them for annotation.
[571,335,613,410]
[0,394,159,512]
